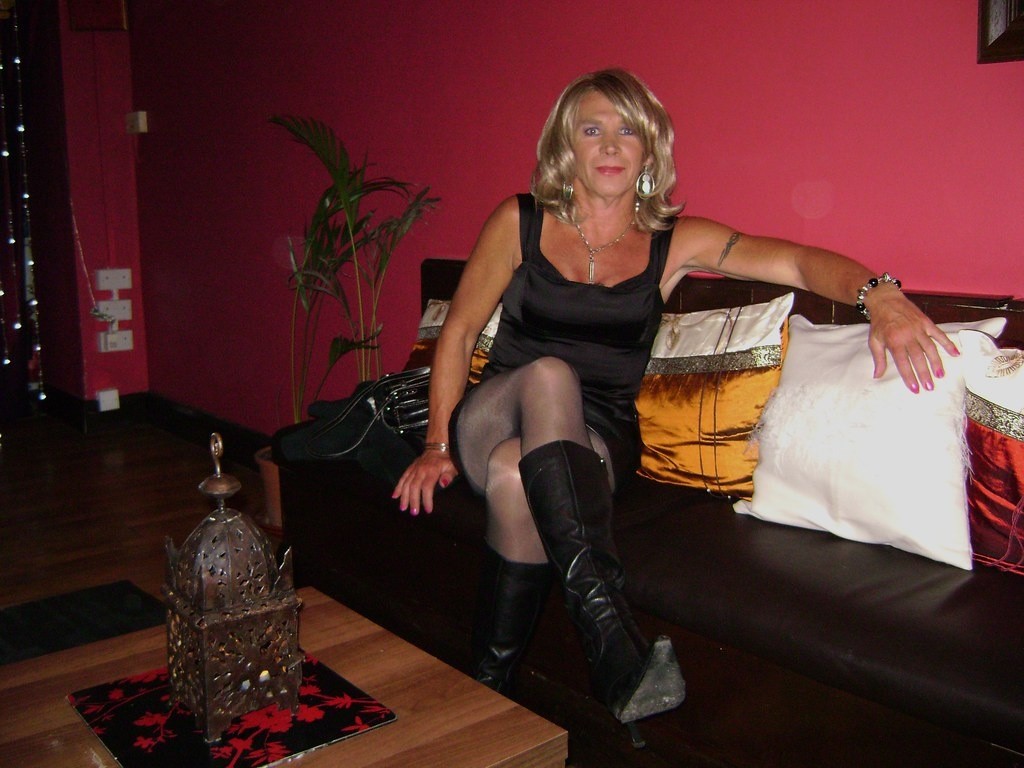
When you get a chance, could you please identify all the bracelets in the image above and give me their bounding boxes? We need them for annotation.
[855,272,902,320]
[424,443,449,451]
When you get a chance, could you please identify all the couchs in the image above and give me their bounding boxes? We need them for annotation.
[271,257,1024,768]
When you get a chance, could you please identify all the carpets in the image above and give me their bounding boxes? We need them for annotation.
[0,579,169,666]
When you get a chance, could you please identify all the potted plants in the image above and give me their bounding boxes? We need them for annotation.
[254,117,440,526]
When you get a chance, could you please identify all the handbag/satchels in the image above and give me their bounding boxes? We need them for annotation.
[287,362,438,465]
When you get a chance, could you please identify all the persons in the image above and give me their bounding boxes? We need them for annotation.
[392,69,961,751]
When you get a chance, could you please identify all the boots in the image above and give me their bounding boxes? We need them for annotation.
[517,438,688,748]
[462,538,556,700]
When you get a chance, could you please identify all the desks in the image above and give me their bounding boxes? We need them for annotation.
[0,586,568,768]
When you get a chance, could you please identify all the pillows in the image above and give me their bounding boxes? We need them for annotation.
[958,330,1024,576]
[635,291,794,501]
[732,315,1005,571]
[412,299,502,385]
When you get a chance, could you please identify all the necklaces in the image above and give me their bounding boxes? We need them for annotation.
[563,202,641,284]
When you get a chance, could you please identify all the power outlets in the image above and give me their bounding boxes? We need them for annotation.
[97,299,133,321]
[97,268,132,289]
[97,389,120,413]
[99,330,134,352]
